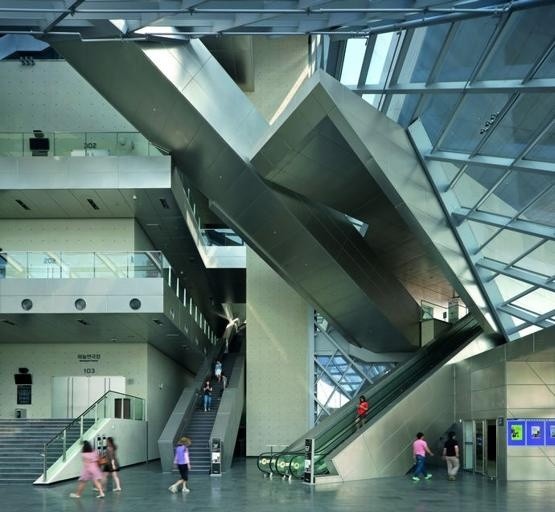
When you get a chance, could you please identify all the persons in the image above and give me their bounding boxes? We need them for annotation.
[411,432,435,482]
[166,437,193,495]
[92,437,124,493]
[215,360,222,384]
[0,247,9,279]
[355,395,369,431]
[441,431,462,482]
[202,379,213,413]
[68,439,107,501]
[218,374,228,402]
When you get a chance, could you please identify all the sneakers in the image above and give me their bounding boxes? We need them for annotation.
[412,474,432,481]
[449,475,456,480]
[169,485,189,493]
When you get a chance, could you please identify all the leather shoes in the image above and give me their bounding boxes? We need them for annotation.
[97,494,104,498]
[70,493,80,498]
[94,486,121,492]
